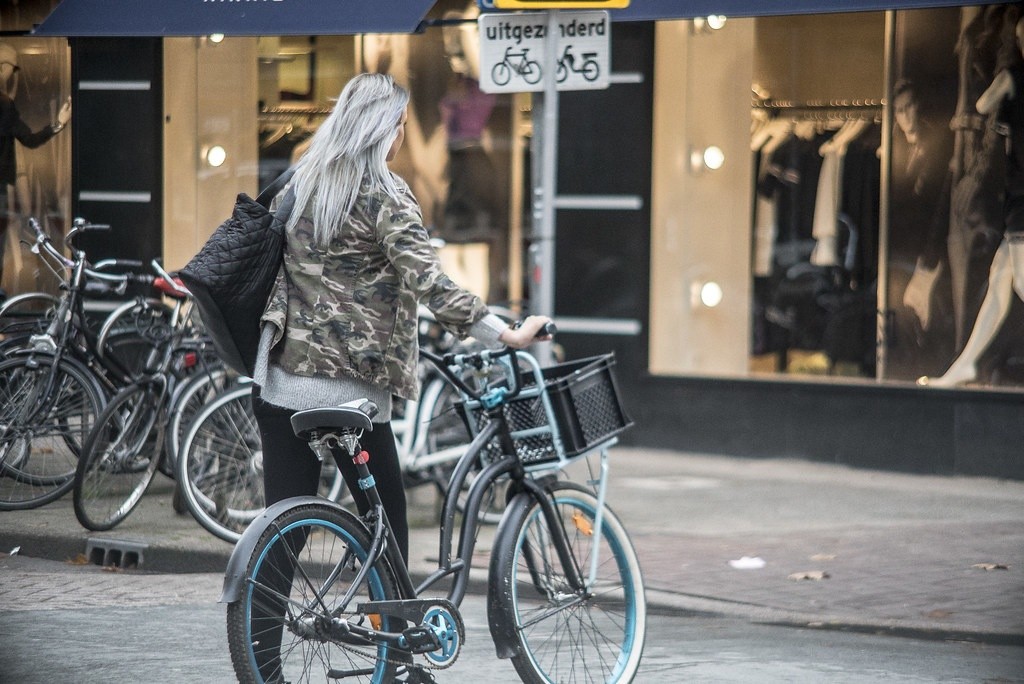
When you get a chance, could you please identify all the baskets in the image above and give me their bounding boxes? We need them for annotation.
[453,352,634,471]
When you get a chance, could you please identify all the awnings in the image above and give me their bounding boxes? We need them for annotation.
[31,0,434,37]
[474,0,1011,24]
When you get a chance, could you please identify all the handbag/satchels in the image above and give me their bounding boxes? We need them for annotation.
[178,164,297,379]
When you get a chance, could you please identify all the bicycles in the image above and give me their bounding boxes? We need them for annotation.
[2,213,562,547]
[213,320,650,684]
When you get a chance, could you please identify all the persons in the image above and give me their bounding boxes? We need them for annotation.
[250,73,553,684]
[435,54,494,241]
[915,18,1024,387]
[888,77,956,380]
[0,40,72,308]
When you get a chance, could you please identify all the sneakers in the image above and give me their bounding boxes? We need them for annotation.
[379,663,438,684]
